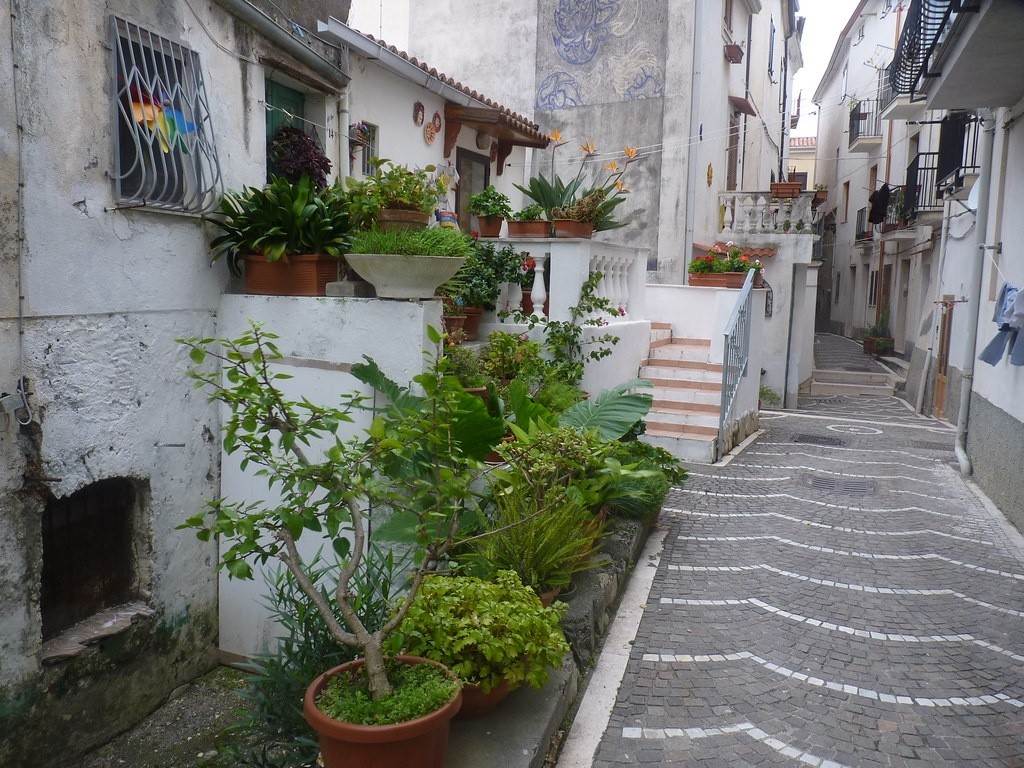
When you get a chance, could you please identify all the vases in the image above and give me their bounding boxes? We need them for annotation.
[521,291,549,317]
[443,316,468,345]
[724,271,762,289]
[688,272,726,287]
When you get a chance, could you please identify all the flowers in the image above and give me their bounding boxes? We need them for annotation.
[688,245,728,273]
[479,329,545,379]
[512,127,649,233]
[543,270,625,387]
[443,295,468,316]
[521,258,550,292]
[722,240,765,272]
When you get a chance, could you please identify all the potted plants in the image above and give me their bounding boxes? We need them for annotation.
[198,172,356,297]
[463,184,513,238]
[551,185,608,239]
[443,346,488,403]
[485,380,517,462]
[566,456,659,569]
[770,167,803,197]
[507,204,553,239]
[725,39,747,64]
[175,318,473,768]
[814,184,829,200]
[847,98,867,120]
[380,566,572,720]
[458,461,616,608]
[857,296,895,357]
[344,221,476,301]
[457,276,501,342]
[344,156,448,236]
[893,190,908,230]
